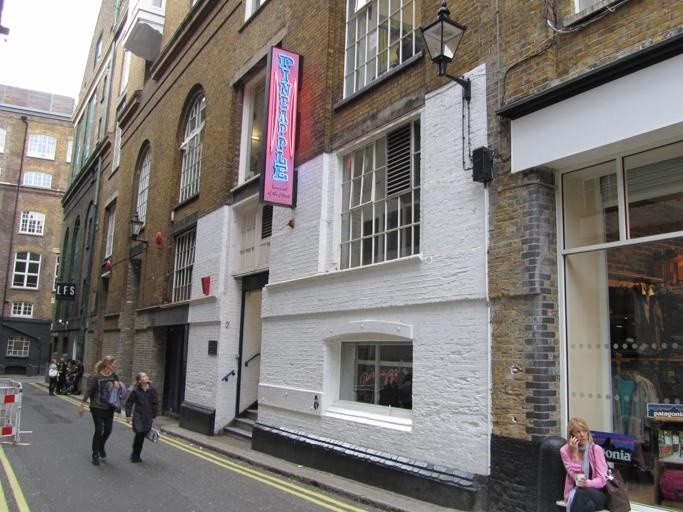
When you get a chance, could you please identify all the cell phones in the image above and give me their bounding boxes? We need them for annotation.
[569,433,576,438]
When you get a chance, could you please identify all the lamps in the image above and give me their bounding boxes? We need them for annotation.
[127,212,149,245]
[418,2,472,101]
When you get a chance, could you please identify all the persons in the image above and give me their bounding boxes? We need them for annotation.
[80,355,120,465]
[125,372,158,463]
[48,358,84,395]
[560,417,608,512]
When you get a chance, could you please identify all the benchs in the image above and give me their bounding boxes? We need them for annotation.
[555,498,680,512]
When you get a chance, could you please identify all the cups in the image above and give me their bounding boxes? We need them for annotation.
[575,471,584,487]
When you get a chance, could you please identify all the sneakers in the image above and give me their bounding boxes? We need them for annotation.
[130,455,143,463]
[92,447,107,465]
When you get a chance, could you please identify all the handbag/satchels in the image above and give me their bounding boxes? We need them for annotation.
[48,368,60,377]
[658,469,683,501]
[605,469,631,512]
[145,428,160,444]
